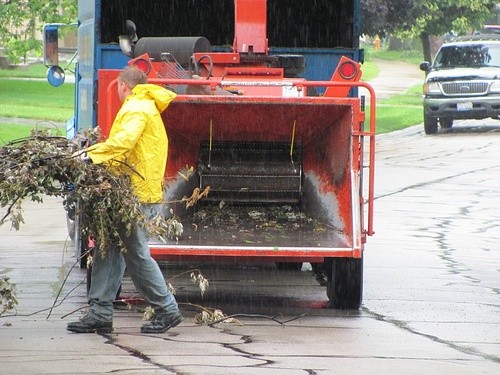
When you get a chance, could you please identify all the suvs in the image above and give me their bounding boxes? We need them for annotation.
[419,38,500,135]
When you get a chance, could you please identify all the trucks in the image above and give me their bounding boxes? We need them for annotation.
[44,1,376,311]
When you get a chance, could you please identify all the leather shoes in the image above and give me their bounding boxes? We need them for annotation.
[66,316,112,332]
[141,310,182,333]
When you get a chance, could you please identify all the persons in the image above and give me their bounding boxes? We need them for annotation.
[66,67,177,333]
